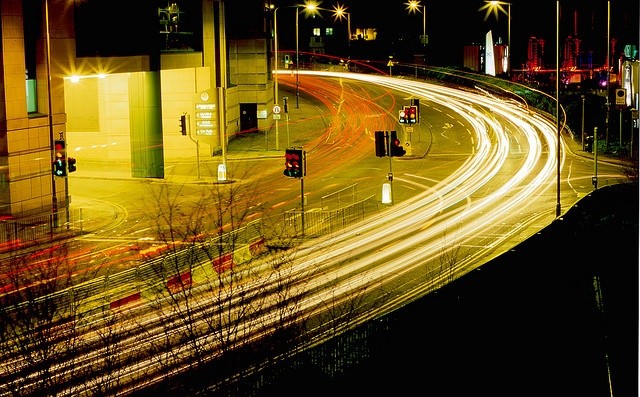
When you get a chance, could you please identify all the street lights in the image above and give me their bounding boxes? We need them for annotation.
[295,4,347,108]
[410,1,426,52]
[274,3,316,151]
[491,0,510,47]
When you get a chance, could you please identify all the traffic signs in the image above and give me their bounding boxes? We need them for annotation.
[190,89,221,147]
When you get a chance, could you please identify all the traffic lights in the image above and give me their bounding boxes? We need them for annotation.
[68,157,76,173]
[403,106,410,124]
[284,148,293,177]
[390,131,399,156]
[398,110,405,124]
[179,115,186,135]
[291,150,301,176]
[409,106,418,124]
[54,140,66,177]
